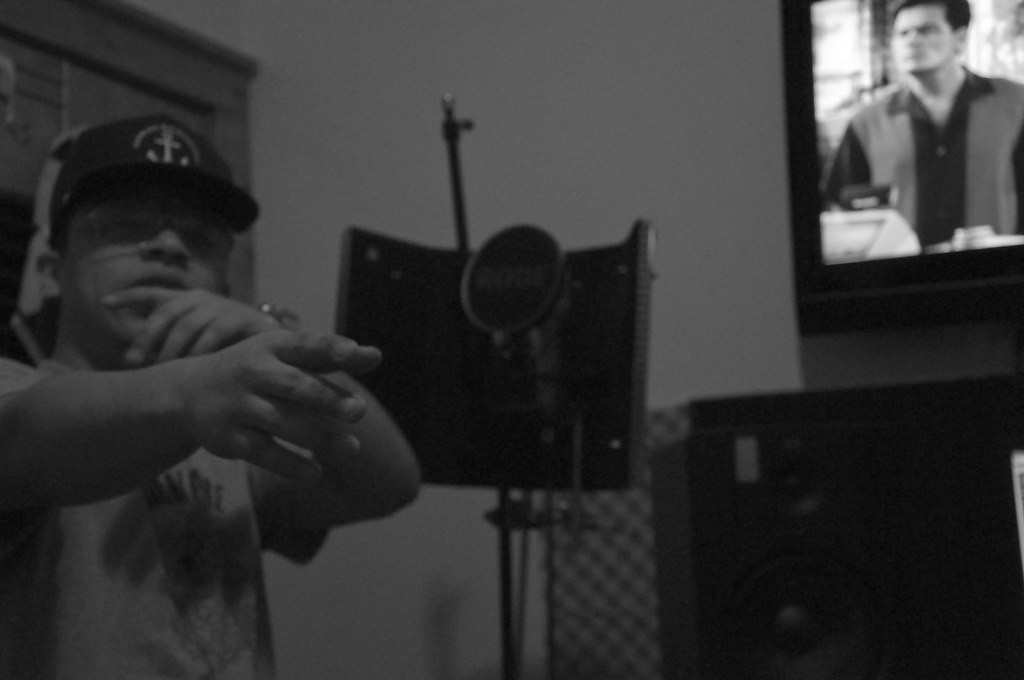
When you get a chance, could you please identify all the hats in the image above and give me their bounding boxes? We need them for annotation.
[51,114,260,240]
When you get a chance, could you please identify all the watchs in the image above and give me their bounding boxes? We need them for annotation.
[255,296,303,331]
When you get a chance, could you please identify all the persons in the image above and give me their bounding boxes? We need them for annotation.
[823,0,1024,253]
[0,131,420,680]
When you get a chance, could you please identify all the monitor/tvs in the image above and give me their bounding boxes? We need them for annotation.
[781,0,1024,335]
